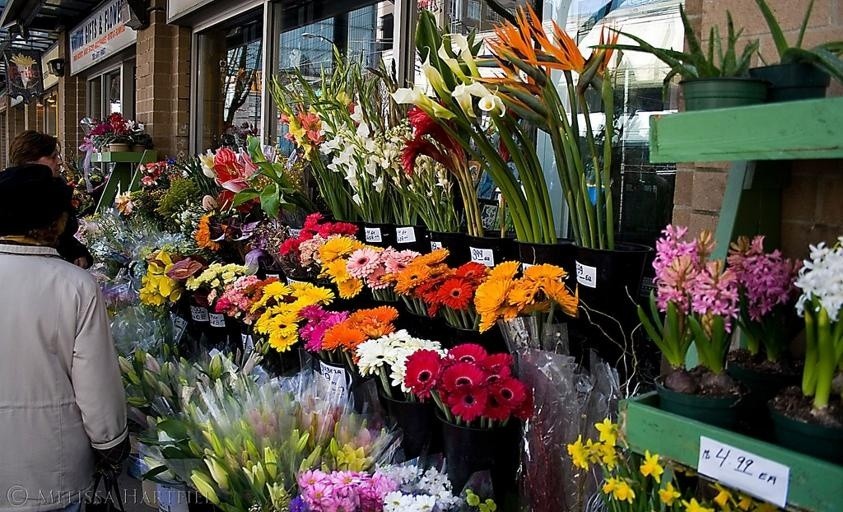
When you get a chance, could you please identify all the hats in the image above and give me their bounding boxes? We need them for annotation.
[0,164,71,237]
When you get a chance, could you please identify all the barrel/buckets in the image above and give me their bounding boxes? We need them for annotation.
[166,211,655,496]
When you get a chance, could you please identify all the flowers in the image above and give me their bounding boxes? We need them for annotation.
[61,2,843,512]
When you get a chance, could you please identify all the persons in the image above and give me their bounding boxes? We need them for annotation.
[8,129,94,270]
[0,163,132,512]
